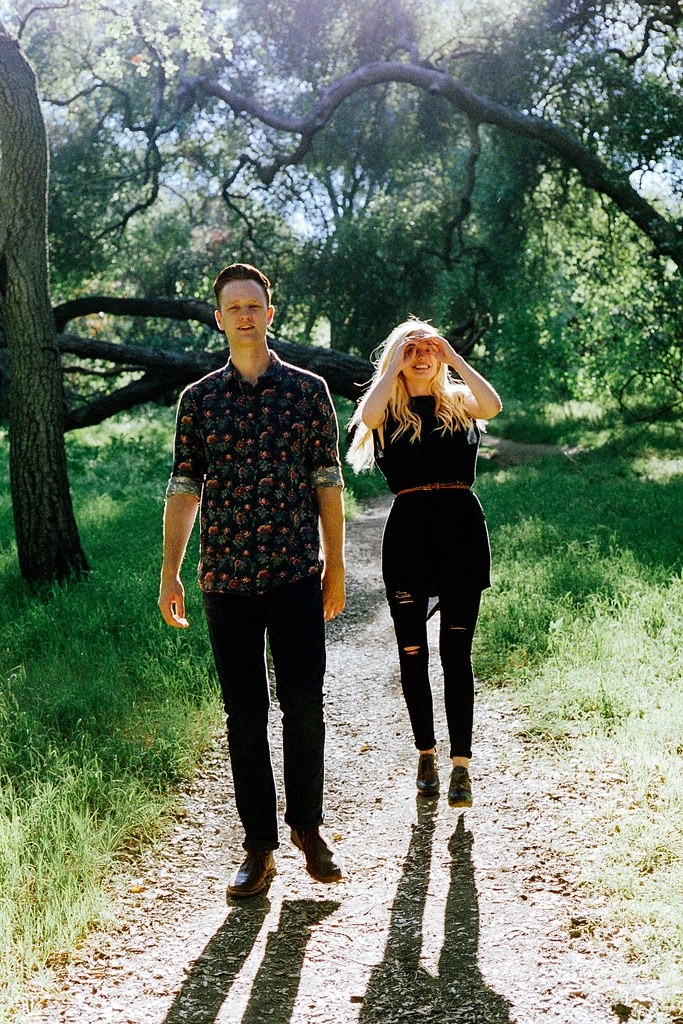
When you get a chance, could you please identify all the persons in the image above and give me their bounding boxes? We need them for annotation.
[343,314,503,810]
[157,260,348,899]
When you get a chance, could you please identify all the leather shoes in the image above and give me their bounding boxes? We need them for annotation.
[227,849,277,896]
[289,825,343,883]
[446,764,473,808]
[417,748,441,795]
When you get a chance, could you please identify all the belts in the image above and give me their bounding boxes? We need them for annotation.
[395,480,472,497]
